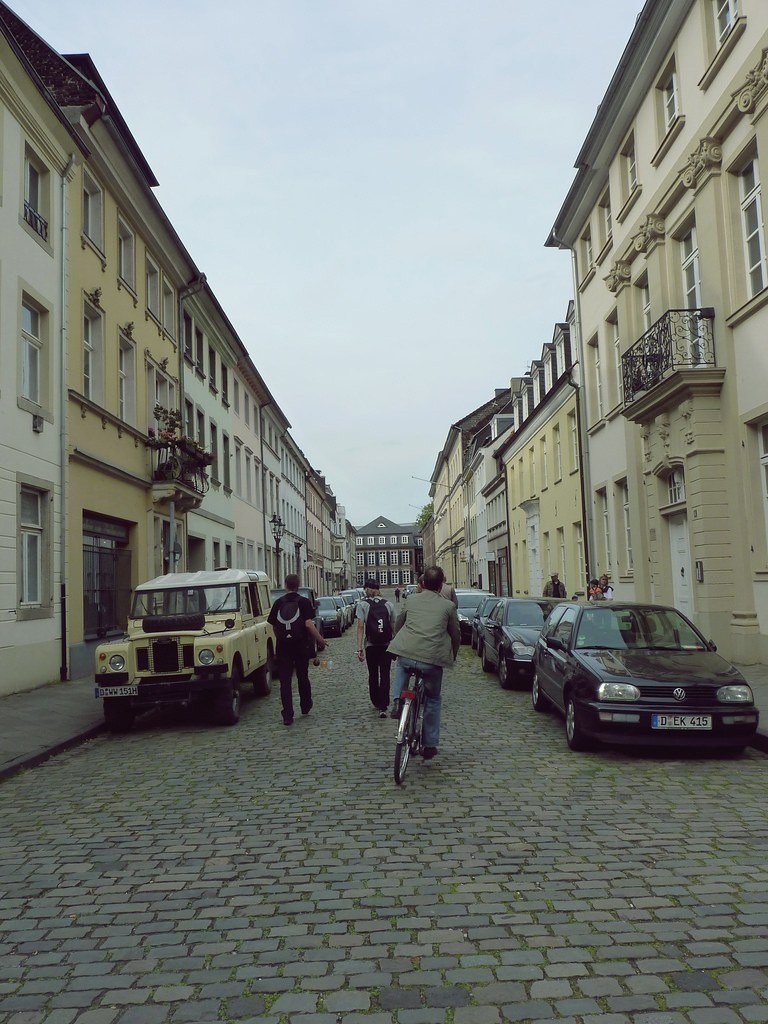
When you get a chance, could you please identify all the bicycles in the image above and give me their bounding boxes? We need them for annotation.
[393,665,426,785]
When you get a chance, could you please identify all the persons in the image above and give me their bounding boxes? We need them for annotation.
[543,572,567,599]
[354,567,460,759]
[267,574,328,725]
[588,575,614,603]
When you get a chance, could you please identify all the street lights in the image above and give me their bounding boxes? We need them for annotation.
[269,512,285,590]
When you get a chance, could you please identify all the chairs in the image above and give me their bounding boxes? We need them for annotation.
[630,616,663,642]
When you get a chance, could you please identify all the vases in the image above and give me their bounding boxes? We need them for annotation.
[166,440,174,447]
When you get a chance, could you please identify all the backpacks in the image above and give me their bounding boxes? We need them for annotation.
[363,599,392,646]
[274,595,308,645]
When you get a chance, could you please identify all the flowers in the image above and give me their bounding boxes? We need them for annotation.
[160,430,174,438]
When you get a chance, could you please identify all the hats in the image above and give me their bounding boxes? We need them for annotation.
[548,571,559,577]
[363,579,379,589]
[590,579,598,585]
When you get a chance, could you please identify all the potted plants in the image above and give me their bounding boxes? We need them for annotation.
[149,404,214,491]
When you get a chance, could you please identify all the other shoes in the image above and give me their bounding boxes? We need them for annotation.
[301,699,314,714]
[379,710,386,718]
[390,699,402,718]
[284,719,294,725]
[423,747,438,759]
[376,708,380,714]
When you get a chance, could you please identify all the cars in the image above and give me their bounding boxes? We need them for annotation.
[455,588,496,645]
[531,601,760,758]
[470,598,512,656]
[480,598,586,690]
[339,595,357,616]
[333,597,352,629]
[316,597,345,637]
[403,585,418,597]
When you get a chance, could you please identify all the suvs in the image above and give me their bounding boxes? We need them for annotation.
[92,569,277,732]
[269,587,325,658]
[339,587,367,605]
